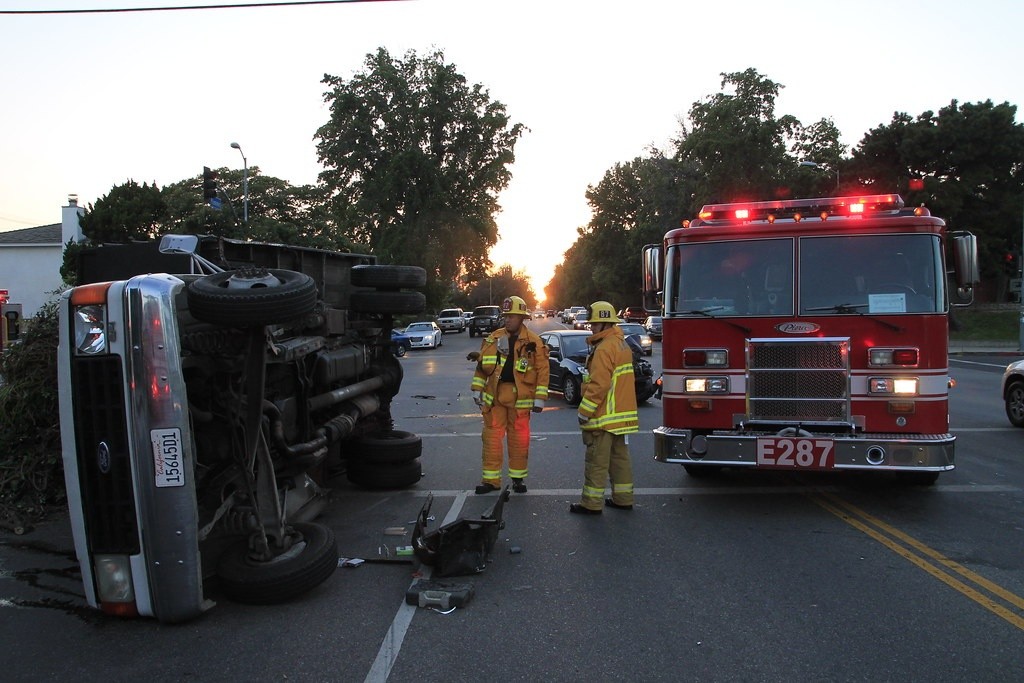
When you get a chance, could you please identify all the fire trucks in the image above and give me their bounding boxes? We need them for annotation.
[642,193,979,488]
[0,288,26,361]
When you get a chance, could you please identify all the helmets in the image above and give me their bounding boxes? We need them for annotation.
[585,301,620,322]
[501,296,530,315]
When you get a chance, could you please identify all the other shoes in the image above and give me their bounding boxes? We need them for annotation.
[570,502,603,516]
[511,476,527,493]
[604,497,632,510]
[475,483,500,495]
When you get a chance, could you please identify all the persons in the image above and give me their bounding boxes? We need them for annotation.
[569,301,641,515]
[471,296,550,494]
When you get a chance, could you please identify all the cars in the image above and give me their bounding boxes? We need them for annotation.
[537,330,660,406]
[390,329,411,358]
[535,311,545,319]
[463,312,475,326]
[557,306,592,331]
[616,309,624,319]
[623,307,648,323]
[640,315,663,339]
[1001,360,1024,429]
[617,323,652,356]
[55,235,426,627]
[401,322,443,349]
[524,309,532,321]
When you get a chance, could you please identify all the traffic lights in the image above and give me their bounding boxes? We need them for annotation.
[204,166,217,205]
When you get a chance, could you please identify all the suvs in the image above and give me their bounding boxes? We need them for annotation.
[469,306,505,339]
[436,308,466,334]
[547,310,554,318]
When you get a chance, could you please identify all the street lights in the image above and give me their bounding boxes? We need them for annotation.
[231,142,247,222]
[797,161,840,190]
